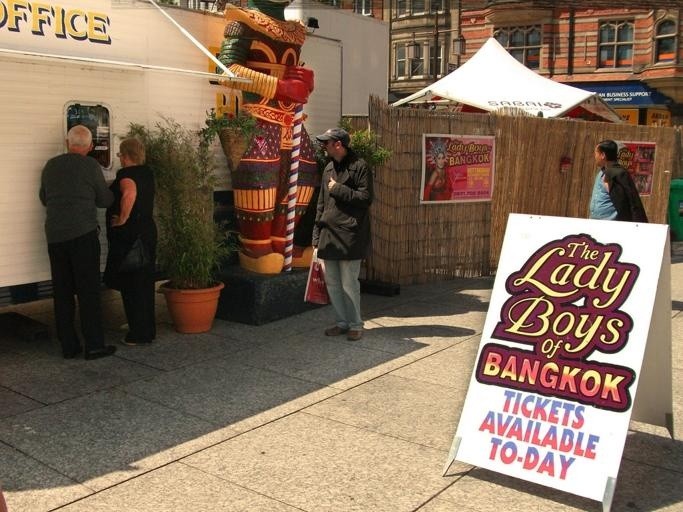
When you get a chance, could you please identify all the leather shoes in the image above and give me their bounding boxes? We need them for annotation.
[66,345,116,360]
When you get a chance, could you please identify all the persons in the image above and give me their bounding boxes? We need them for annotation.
[589,140,649,222]
[311,127,374,341]
[216,0,321,276]
[39,124,115,360]
[105,138,159,346]
[424,143,454,201]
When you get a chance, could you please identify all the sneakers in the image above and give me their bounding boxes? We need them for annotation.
[326,326,362,339]
[121,334,152,346]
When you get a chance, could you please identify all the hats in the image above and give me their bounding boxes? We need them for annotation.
[316,128,350,145]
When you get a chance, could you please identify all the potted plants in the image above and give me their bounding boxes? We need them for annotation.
[199,109,266,173]
[112,115,225,333]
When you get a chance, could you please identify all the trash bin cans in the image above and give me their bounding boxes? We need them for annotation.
[668,179,683,241]
